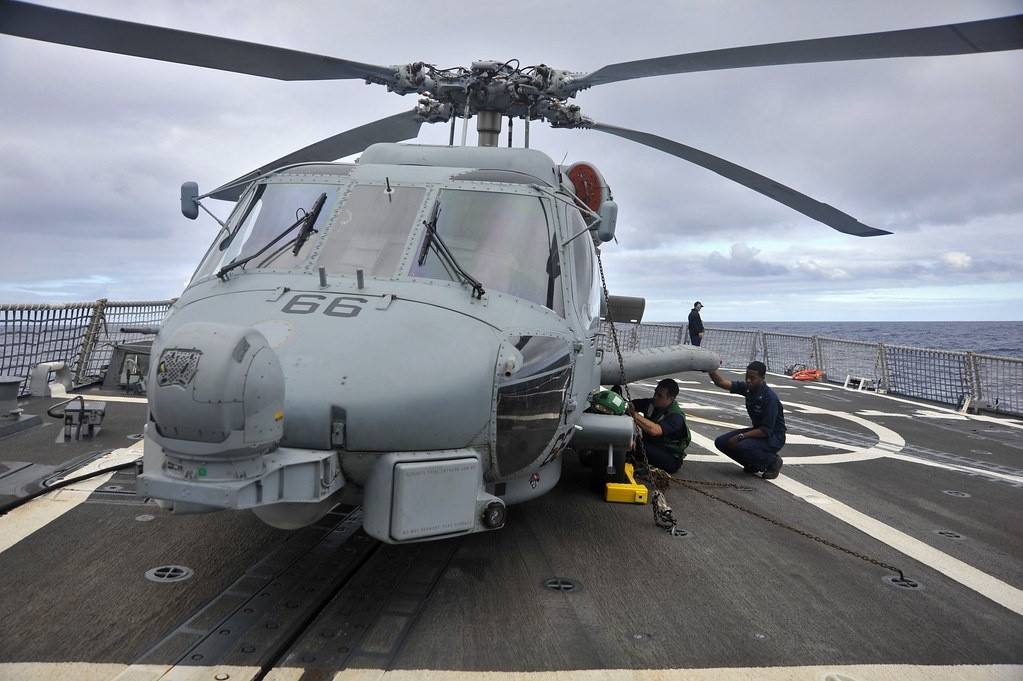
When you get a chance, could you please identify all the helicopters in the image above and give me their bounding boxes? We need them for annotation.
[0,0,1023,551]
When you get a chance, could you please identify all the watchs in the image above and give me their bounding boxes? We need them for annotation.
[738,433,744,440]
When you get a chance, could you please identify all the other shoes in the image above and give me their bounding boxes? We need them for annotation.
[743,465,759,473]
[762,455,783,480]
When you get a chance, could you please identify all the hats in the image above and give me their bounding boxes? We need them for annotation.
[694,302,703,307]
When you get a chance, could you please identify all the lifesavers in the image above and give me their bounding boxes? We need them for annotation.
[792,369,823,381]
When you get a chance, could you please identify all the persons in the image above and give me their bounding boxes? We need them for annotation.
[627,378,691,473]
[709,361,787,479]
[688,301,705,346]
